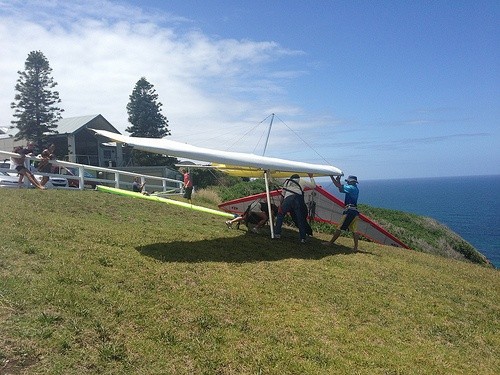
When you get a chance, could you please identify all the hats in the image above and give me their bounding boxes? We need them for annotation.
[345,175,359,184]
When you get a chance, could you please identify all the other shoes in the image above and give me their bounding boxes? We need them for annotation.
[251,228,261,234]
[275,234,281,239]
[225,221,233,228]
[301,239,304,243]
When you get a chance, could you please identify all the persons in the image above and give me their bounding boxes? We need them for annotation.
[323,176,360,250]
[132,176,150,196]
[178,168,193,203]
[40,144,55,186]
[225,202,278,233]
[12,146,47,189]
[274,174,316,242]
[18,141,34,184]
